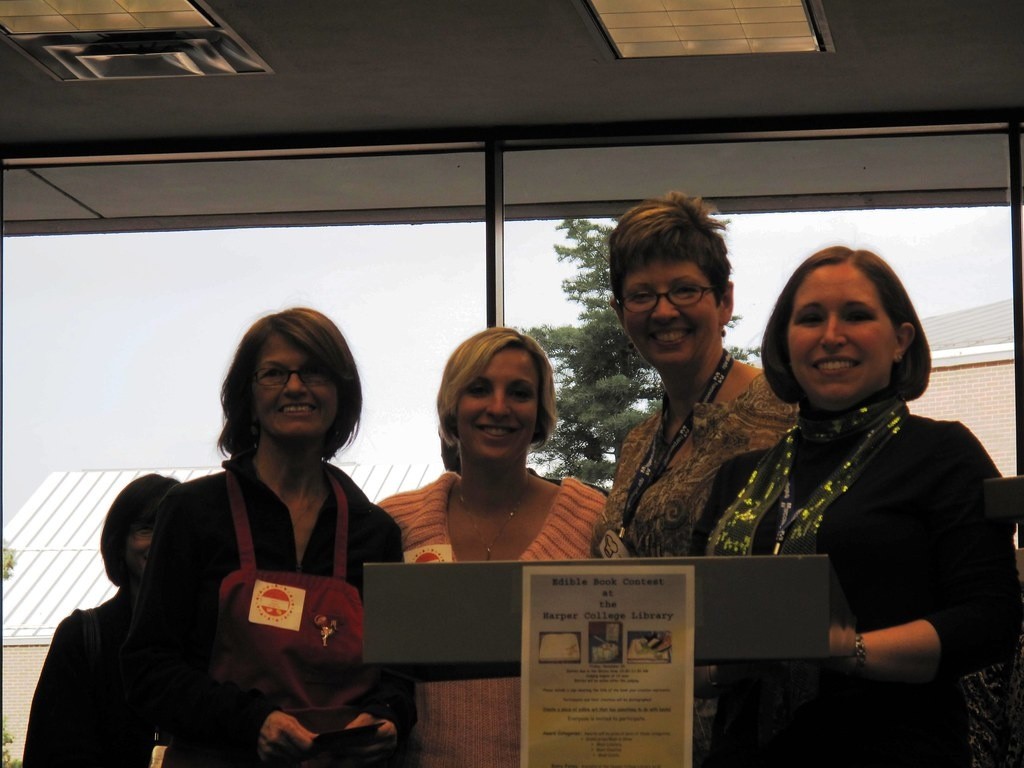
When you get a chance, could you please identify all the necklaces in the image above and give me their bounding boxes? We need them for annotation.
[293,485,326,531]
[458,472,529,560]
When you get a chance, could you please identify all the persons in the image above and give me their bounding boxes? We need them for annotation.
[118,309,403,768]
[375,330,619,768]
[22,474,181,768]
[590,190,799,555]
[690,246,1024,768]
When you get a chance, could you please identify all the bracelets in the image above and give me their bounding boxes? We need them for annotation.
[853,635,866,677]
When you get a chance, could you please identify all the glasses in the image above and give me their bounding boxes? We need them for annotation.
[615,283,719,315]
[251,361,338,387]
